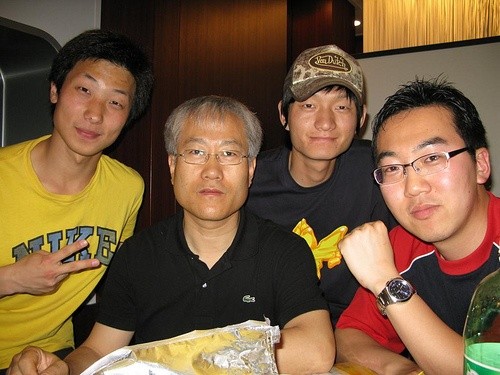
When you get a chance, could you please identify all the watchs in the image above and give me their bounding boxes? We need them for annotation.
[377,277,415,318]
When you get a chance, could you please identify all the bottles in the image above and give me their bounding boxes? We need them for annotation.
[462,247,500,375]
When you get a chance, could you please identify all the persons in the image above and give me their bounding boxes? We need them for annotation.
[335,72,500,375]
[0,94,337,375]
[0,29,146,375]
[243,44,400,328]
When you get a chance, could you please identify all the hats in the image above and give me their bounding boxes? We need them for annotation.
[280,45,366,108]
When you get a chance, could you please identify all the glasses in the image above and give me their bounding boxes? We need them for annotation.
[175,147,249,166]
[373,142,475,188]
